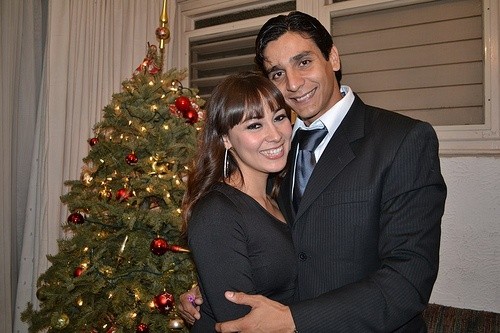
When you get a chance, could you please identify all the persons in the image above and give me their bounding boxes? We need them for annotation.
[178,11,447,333]
[189,69,300,333]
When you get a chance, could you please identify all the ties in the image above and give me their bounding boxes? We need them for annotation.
[293,126,329,214]
